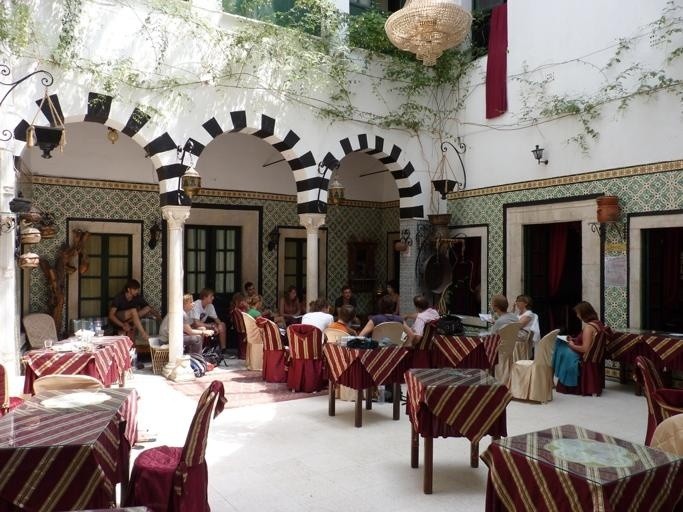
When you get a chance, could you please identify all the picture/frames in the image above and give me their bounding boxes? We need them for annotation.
[450,223,488,328]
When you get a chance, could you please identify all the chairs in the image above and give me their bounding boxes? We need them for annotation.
[127,317,160,353]
[0,365,225,512]
[23,312,58,348]
[635,354,682,446]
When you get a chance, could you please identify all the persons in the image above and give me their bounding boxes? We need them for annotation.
[398,295,439,348]
[159,294,214,371]
[550,302,604,388]
[107,278,162,343]
[188,288,236,360]
[230,281,414,401]
[509,294,542,360]
[487,294,518,336]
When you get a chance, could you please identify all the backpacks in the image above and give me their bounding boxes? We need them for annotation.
[202,346,228,368]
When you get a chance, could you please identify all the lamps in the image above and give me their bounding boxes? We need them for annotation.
[531,145,548,166]
[268,226,280,251]
[384,0,473,68]
[316,160,345,212]
[147,220,160,250]
[108,127,119,146]
[177,136,201,206]
[395,229,412,251]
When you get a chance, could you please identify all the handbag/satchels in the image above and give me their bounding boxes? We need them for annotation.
[430,316,465,335]
[190,352,207,377]
[348,337,379,348]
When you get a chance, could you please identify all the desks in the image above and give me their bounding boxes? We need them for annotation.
[606,326,683,396]
[24,335,130,389]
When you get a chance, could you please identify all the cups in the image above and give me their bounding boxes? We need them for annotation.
[44,340,53,353]
[74,318,105,340]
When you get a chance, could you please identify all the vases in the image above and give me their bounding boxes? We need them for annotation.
[595,195,619,222]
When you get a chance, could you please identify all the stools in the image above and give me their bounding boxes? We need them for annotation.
[149,338,169,375]
